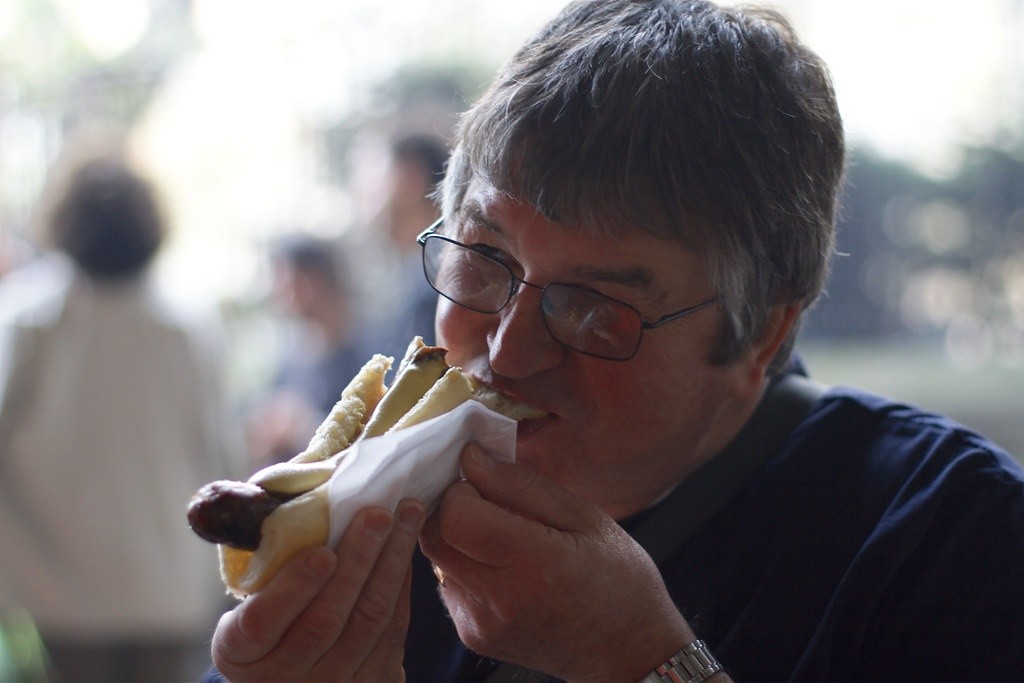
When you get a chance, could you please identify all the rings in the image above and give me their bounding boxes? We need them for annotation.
[433,564,449,585]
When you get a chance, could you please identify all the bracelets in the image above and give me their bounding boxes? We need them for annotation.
[640,639,724,683]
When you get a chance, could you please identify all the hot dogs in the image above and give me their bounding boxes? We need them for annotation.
[186,333,547,599]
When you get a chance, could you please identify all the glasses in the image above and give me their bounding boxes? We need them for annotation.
[417,216,720,362]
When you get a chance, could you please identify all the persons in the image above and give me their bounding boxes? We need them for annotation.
[0,158,250,683]
[244,236,367,465]
[378,133,454,386]
[201,0,1024,683]
